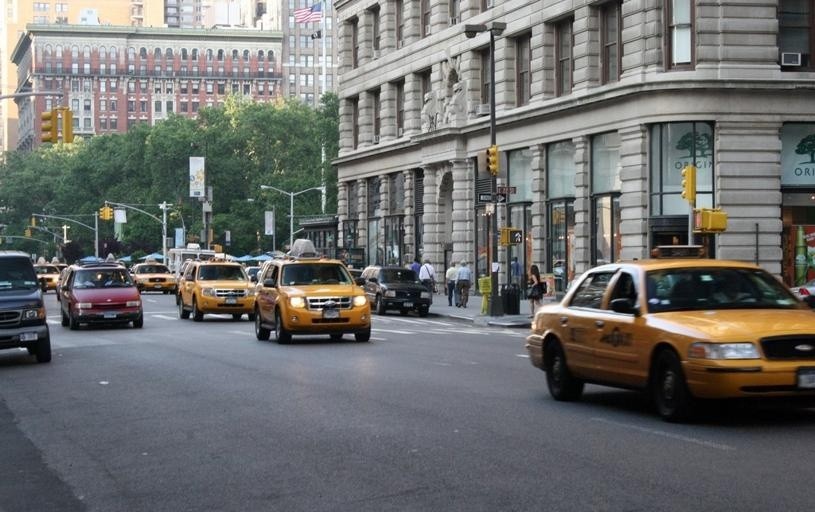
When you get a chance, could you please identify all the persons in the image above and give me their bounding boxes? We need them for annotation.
[527,266,542,318]
[321,266,335,280]
[411,259,471,308]
[511,258,523,288]
[711,276,755,303]
[552,262,564,291]
[104,271,123,286]
[74,274,94,286]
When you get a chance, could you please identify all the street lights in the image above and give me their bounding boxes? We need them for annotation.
[460,22,507,316]
[247,198,276,252]
[260,183,325,252]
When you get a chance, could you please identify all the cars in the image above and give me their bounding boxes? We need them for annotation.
[32,254,144,329]
[526,261,815,417]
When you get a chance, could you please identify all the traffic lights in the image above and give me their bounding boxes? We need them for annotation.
[39,106,75,144]
[509,230,523,244]
[681,162,698,208]
[31,217,41,229]
[97,205,114,220]
[486,145,500,176]
[23,229,31,238]
[694,208,728,232]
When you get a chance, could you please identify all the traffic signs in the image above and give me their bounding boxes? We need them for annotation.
[496,186,517,195]
[477,193,506,204]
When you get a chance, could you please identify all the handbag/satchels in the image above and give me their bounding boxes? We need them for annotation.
[528,282,547,294]
[430,275,435,287]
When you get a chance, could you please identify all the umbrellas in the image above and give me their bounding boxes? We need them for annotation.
[80,253,273,261]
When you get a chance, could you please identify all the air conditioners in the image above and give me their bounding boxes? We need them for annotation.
[782,53,802,68]
[475,103,489,116]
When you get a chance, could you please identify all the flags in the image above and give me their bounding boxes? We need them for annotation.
[293,2,321,23]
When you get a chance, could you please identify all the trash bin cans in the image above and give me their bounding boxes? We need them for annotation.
[500,283,521,314]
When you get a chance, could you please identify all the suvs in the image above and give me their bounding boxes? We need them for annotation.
[0,249,52,363]
